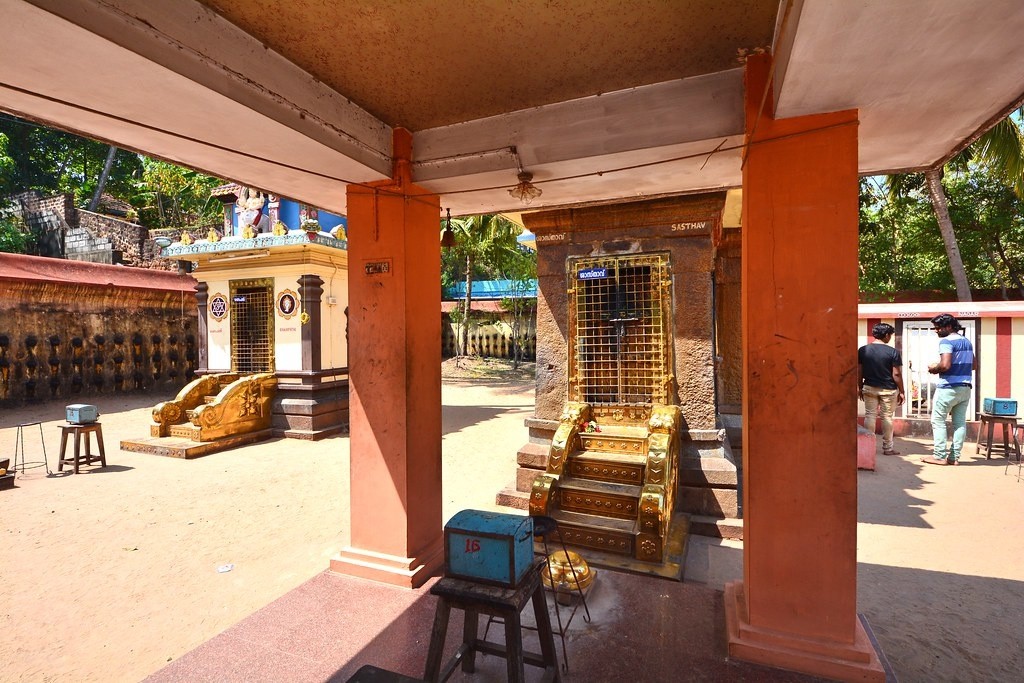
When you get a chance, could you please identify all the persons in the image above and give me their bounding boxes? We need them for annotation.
[859,323,908,456]
[921,315,979,467]
[243,188,266,230]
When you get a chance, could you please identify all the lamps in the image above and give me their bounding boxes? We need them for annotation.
[505,172,545,205]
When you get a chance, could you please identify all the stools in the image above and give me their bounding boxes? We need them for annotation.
[857,422,877,473]
[974,411,1021,461]
[57,422,107,473]
[424,556,561,682]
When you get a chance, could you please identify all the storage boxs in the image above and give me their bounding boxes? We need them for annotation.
[443,509,536,588]
[66,403,98,425]
[983,396,1018,417]
